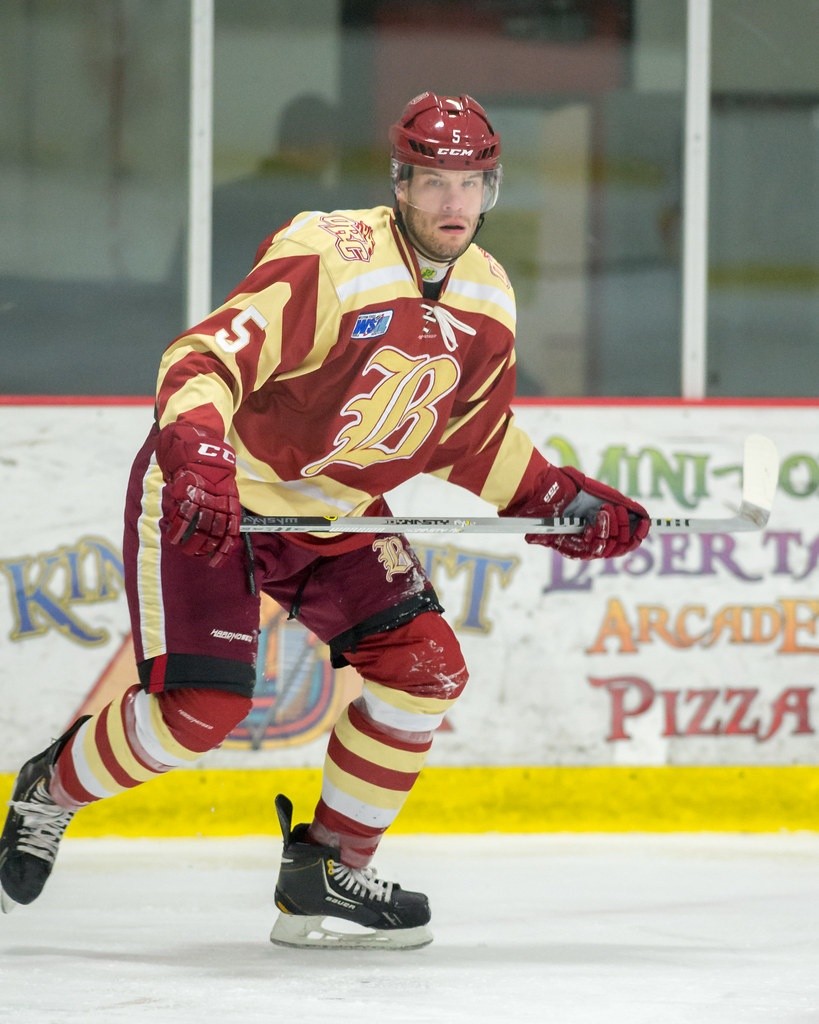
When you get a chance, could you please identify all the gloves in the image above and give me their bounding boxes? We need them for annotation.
[161,438,240,569]
[517,459,653,559]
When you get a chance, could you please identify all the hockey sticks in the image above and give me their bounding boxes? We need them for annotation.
[239,433,779,535]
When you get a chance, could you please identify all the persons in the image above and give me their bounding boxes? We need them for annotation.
[0,90,650,951]
[158,94,345,399]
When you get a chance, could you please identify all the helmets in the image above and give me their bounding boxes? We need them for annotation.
[389,91,501,175]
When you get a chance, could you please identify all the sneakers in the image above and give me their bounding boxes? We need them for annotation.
[272,794,434,950]
[0,714,96,913]
